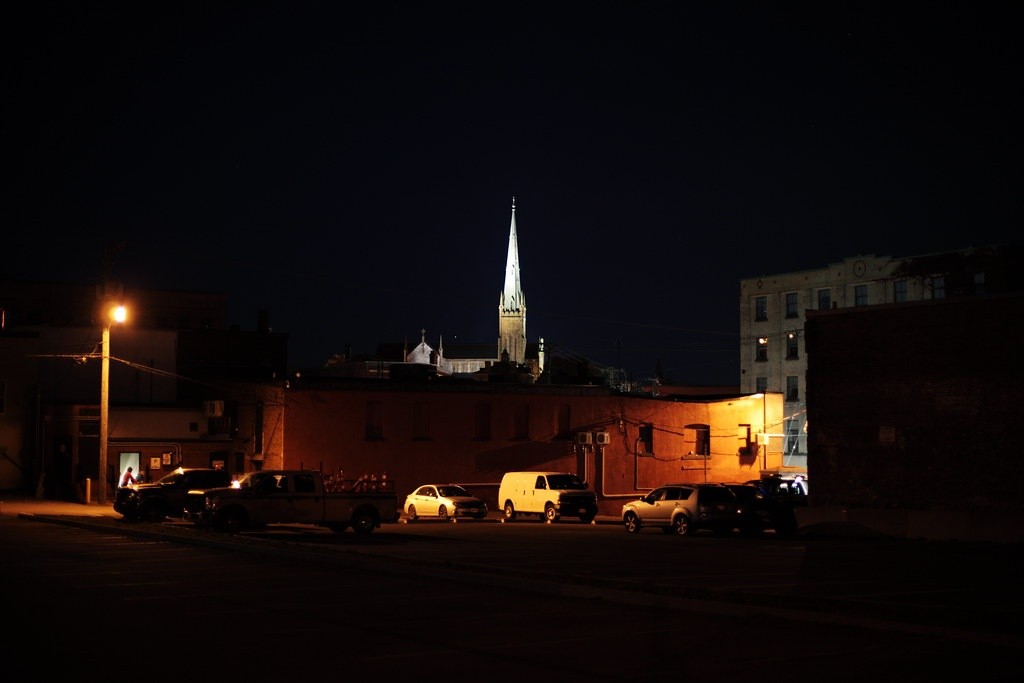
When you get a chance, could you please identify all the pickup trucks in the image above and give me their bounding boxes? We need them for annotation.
[200,471,403,538]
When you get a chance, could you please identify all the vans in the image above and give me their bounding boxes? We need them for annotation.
[497,472,599,525]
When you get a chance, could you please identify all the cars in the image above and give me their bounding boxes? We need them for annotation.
[403,483,487,523]
[723,485,804,542]
[114,466,234,522]
[742,474,808,508]
[621,483,736,536]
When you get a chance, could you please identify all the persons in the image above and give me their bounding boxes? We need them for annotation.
[121,466,138,488]
[135,466,145,484]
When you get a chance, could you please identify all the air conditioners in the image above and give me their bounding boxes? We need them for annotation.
[574,431,593,445]
[595,431,610,445]
